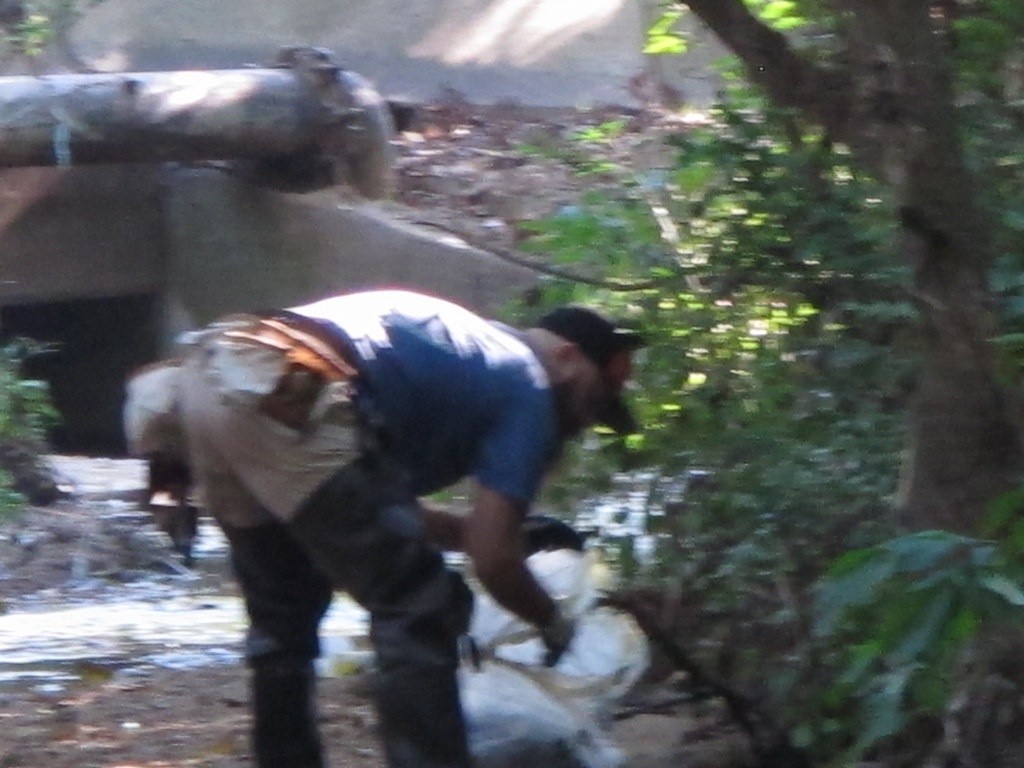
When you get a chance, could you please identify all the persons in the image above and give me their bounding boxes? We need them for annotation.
[177,288,643,768]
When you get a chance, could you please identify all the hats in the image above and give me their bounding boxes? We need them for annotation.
[536,308,639,436]
